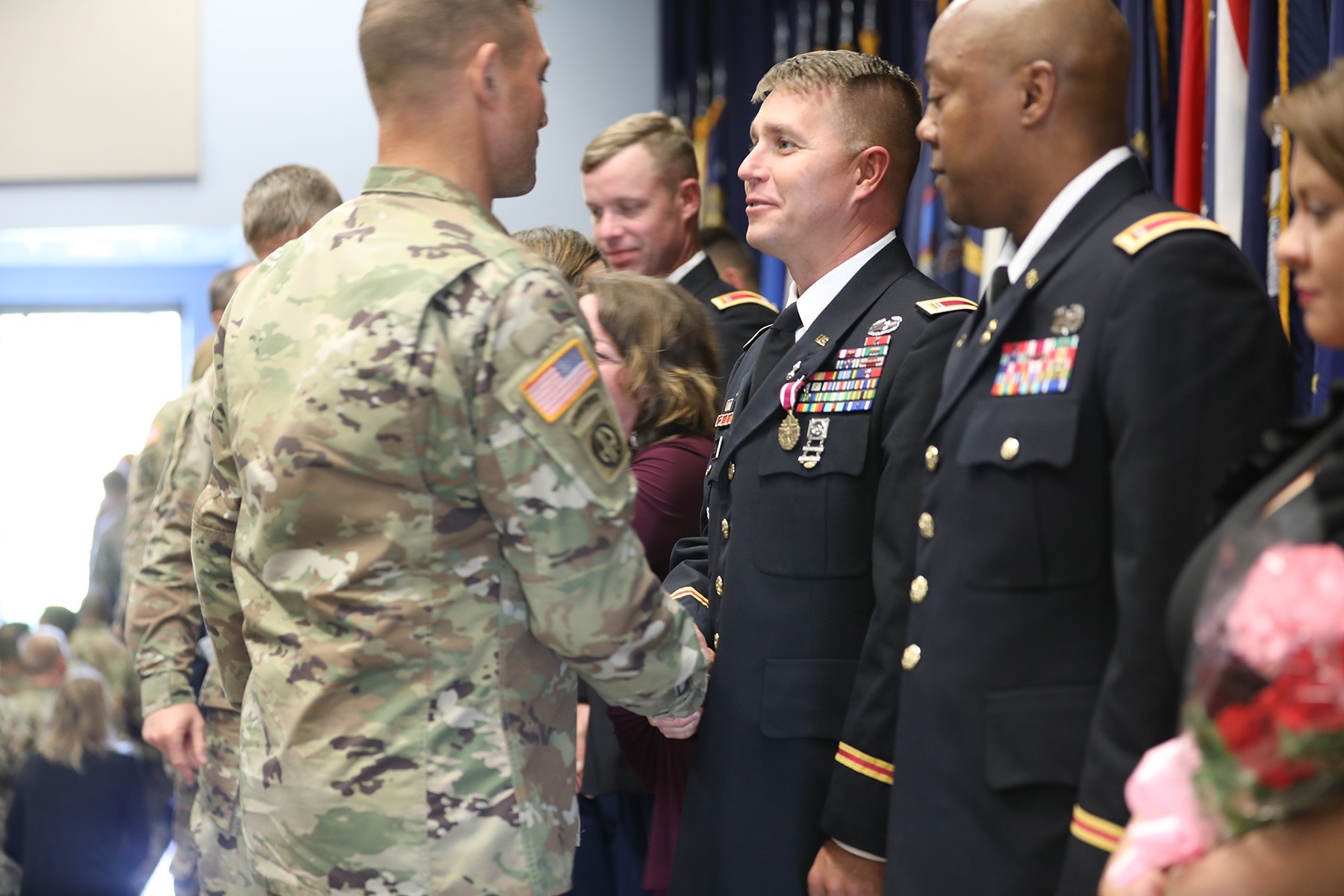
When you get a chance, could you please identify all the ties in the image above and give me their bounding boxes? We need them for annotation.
[965,266,1008,345]
[749,302,804,399]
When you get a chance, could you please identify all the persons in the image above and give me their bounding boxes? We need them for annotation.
[1096,58,1344,896]
[0,165,345,896]
[503,106,778,895]
[884,1,1296,896]
[651,52,979,895]
[190,0,714,896]
